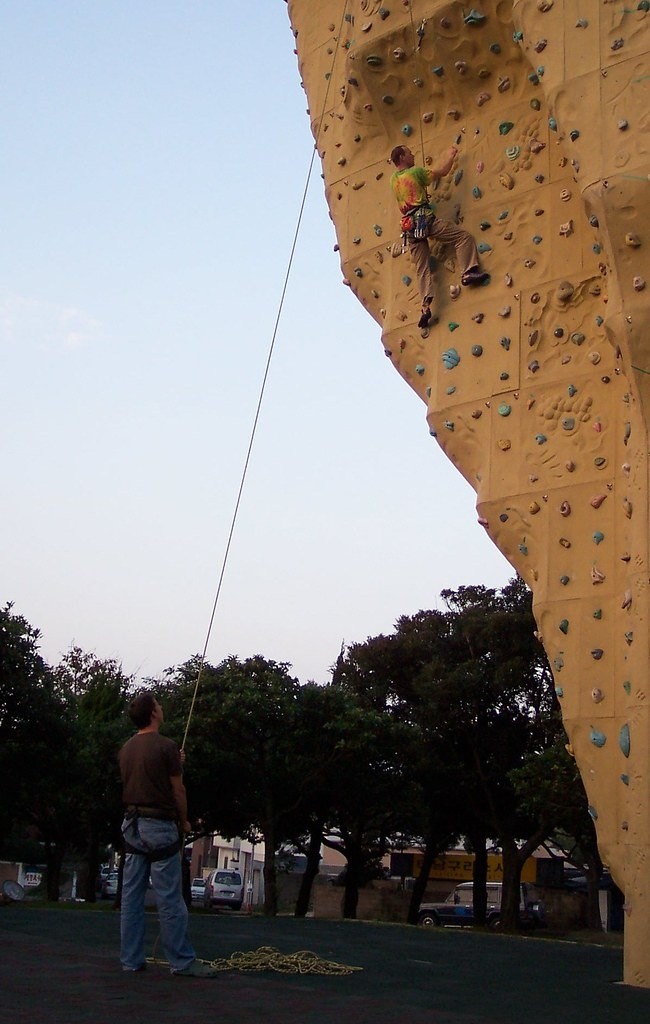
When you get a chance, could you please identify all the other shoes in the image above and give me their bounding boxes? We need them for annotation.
[172,960,218,978]
[122,962,160,977]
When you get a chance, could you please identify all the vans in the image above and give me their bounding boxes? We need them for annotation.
[204,868,244,909]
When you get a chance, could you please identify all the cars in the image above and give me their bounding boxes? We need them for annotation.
[96,866,118,883]
[191,878,206,901]
[101,872,121,898]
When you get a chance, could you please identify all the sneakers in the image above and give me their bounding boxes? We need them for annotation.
[418,306,431,328]
[461,266,489,286]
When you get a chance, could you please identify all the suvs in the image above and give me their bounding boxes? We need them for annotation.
[416,882,550,934]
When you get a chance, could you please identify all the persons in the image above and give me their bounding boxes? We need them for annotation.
[390,145,490,328]
[119,693,218,977]
